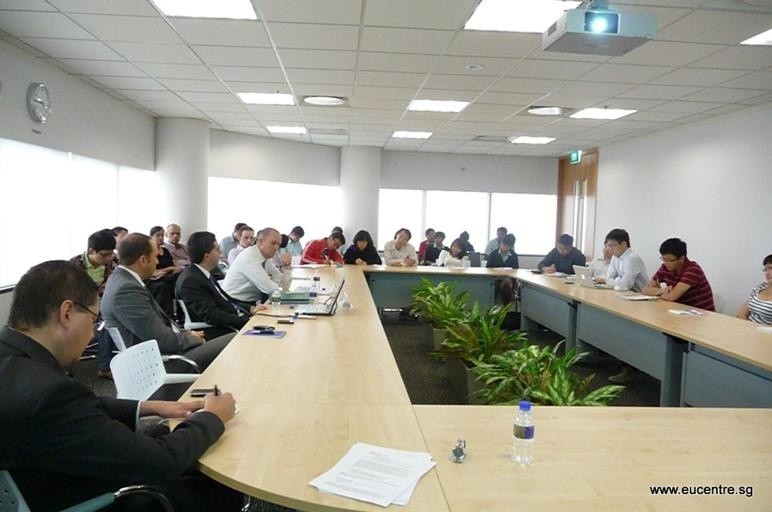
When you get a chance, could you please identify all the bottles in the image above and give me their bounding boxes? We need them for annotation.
[311,269,321,292]
[510,399,537,465]
[287,305,297,321]
[308,286,318,304]
[272,285,281,314]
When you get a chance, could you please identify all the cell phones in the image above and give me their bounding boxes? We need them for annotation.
[278,319,294,324]
[191,389,220,397]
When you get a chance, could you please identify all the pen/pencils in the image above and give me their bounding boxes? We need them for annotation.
[250,331,274,335]
[214,385,220,397]
[325,254,330,261]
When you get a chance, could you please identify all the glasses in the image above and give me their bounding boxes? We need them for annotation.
[74,301,107,332]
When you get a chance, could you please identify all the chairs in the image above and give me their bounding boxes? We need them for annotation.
[1,470,256,511]
[78,273,242,402]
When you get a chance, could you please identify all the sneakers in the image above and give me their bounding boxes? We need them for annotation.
[608,366,641,384]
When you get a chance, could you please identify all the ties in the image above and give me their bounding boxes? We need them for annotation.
[208,276,251,316]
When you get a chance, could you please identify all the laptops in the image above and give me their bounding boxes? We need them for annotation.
[295,277,344,315]
[572,264,614,289]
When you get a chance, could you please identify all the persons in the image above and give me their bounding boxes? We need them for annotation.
[302,226,586,319]
[70,220,303,381]
[1,258,238,511]
[641,238,714,316]
[593,228,649,382]
[735,253,772,327]
[583,240,615,284]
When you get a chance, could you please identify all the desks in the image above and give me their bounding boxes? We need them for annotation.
[360,261,511,320]
[514,269,771,405]
[410,398,771,512]
[168,260,456,512]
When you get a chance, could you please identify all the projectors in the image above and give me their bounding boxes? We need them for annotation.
[543,8,657,56]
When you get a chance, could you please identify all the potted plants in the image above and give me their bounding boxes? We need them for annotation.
[440,300,530,405]
[406,275,473,351]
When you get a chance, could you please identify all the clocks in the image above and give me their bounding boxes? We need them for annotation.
[26,81,53,126]
